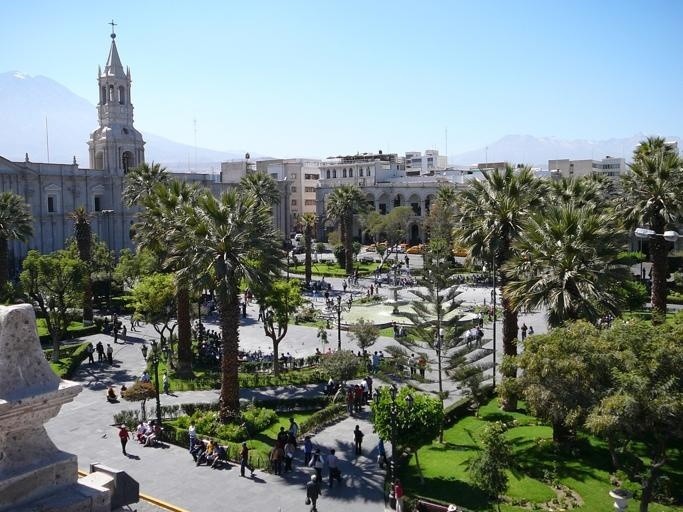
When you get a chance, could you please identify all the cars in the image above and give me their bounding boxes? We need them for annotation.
[294,233,314,243]
[366,241,469,257]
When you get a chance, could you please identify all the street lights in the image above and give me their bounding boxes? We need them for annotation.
[141,340,170,427]
[373,384,413,509]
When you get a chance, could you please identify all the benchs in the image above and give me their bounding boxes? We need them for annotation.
[218,446,229,466]
[155,428,164,444]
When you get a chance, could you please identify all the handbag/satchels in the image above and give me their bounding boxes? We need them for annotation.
[305,498,310,504]
[316,461,323,468]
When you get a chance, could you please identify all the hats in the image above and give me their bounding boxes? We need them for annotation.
[121,424,126,428]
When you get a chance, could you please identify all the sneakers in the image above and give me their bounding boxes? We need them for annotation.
[338,478,343,482]
[329,484,332,488]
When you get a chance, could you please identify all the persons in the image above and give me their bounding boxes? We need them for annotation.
[325,375,383,415]
[455,273,490,286]
[119,420,161,455]
[596,312,636,330]
[395,481,402,512]
[392,321,407,338]
[207,300,220,317]
[270,419,339,512]
[196,322,332,366]
[343,274,352,291]
[88,315,169,402]
[351,349,426,377]
[433,327,446,353]
[303,276,331,300]
[354,425,364,455]
[379,438,388,470]
[370,254,418,294]
[189,422,254,476]
[466,285,504,348]
[522,324,534,340]
[245,286,263,321]
